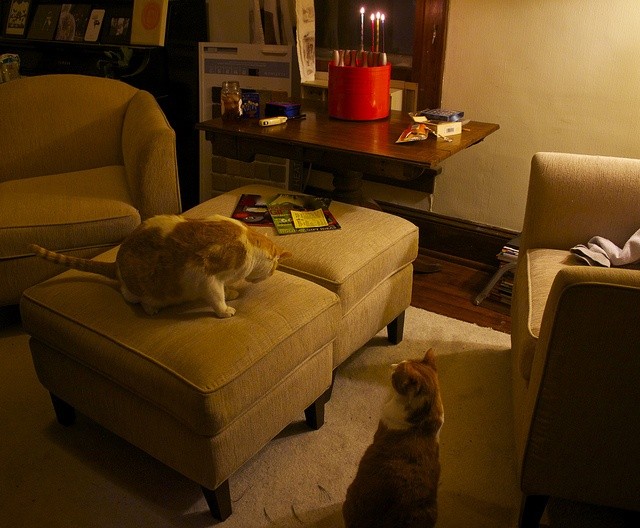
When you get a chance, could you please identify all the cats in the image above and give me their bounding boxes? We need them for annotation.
[28,214,292,317]
[343,347,444,528]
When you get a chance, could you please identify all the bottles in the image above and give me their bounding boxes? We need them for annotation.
[219,80,245,123]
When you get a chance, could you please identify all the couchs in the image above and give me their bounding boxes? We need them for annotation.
[509,151,639,526]
[0,75,185,307]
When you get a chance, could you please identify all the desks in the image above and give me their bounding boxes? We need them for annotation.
[193,96,500,183]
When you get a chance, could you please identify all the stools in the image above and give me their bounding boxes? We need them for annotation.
[174,182,422,402]
[18,241,345,523]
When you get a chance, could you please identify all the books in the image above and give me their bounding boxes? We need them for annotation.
[489,233,520,306]
